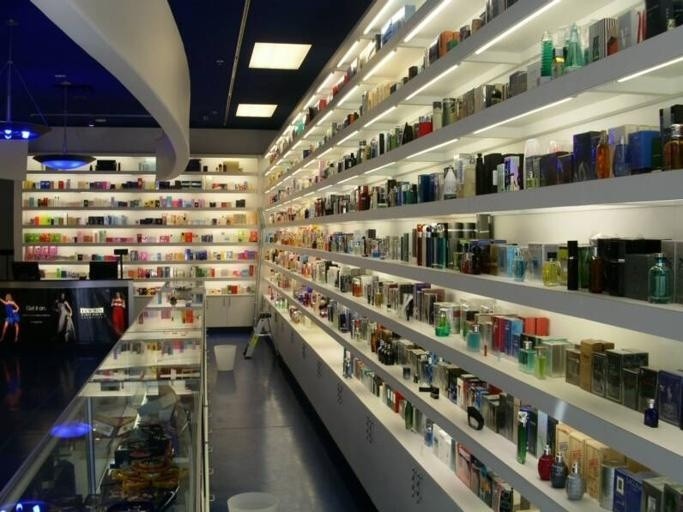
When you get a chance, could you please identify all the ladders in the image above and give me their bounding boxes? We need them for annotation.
[243,312,280,360]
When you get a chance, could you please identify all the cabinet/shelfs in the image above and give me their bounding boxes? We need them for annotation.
[261,2,683,512]
[2,285,208,512]
[28,155,256,330]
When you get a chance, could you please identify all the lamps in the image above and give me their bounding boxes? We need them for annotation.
[0,119,52,145]
[235,103,279,118]
[246,40,313,71]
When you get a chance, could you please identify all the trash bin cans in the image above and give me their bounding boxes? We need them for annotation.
[214,344,237,371]
[227,491,279,512]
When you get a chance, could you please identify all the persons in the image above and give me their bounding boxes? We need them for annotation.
[0,293,19,341]
[110,291,126,334]
[51,292,73,338]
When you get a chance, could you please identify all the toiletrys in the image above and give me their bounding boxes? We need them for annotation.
[258,0,682,508]
[24,154,259,305]
